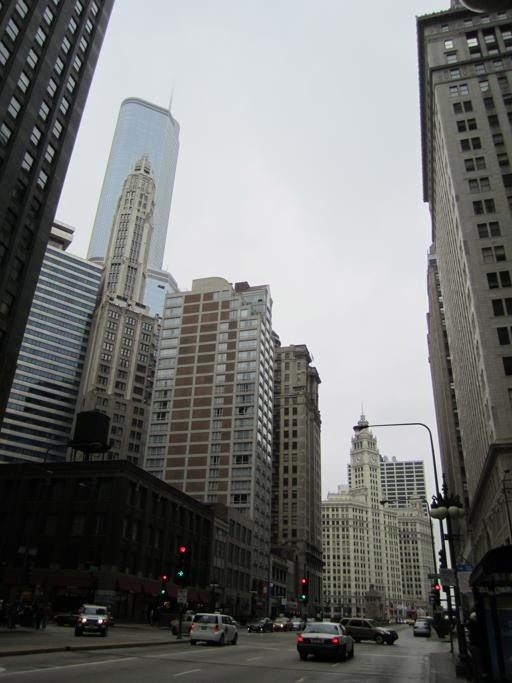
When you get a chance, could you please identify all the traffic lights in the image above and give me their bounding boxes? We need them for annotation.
[160,572,169,595]
[438,548,443,569]
[434,583,440,592]
[299,578,310,604]
[176,540,193,582]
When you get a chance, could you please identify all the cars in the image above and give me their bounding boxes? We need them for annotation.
[399,616,415,625]
[170,613,196,634]
[306,617,316,621]
[295,621,355,660]
[54,606,114,627]
[272,617,293,631]
[290,617,305,630]
[247,615,273,632]
[413,616,430,637]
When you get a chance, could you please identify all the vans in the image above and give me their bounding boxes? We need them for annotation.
[189,612,238,646]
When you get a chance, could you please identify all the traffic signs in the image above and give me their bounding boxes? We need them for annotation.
[427,573,440,579]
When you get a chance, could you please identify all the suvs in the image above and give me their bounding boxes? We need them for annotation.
[337,616,398,645]
[74,603,110,636]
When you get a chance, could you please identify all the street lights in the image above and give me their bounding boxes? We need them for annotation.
[380,498,441,610]
[427,469,476,678]
[353,423,455,656]
[14,441,103,597]
